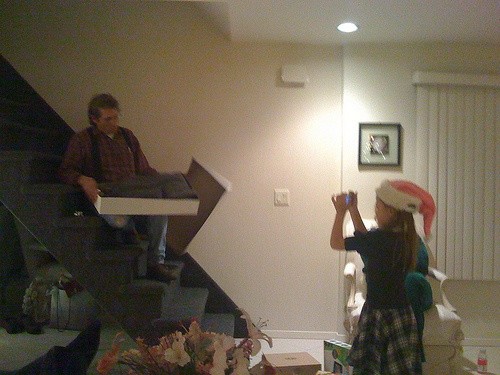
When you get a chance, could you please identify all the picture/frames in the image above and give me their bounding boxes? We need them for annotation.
[358,122,401,166]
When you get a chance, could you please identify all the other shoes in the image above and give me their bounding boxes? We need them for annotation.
[146,266,178,281]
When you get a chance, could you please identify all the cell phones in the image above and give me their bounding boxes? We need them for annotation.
[345,194,354,208]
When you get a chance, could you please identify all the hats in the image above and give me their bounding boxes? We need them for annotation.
[375,179,435,243]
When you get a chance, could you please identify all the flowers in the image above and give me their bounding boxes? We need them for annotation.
[96,307,274,375]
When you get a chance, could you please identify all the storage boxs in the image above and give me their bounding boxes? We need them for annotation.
[94,174,200,215]
[262,352,321,375]
[324,339,354,375]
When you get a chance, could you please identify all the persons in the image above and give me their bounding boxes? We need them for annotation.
[328,179,435,375]
[58,92,178,282]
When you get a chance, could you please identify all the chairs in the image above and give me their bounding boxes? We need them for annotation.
[343,219,465,375]
